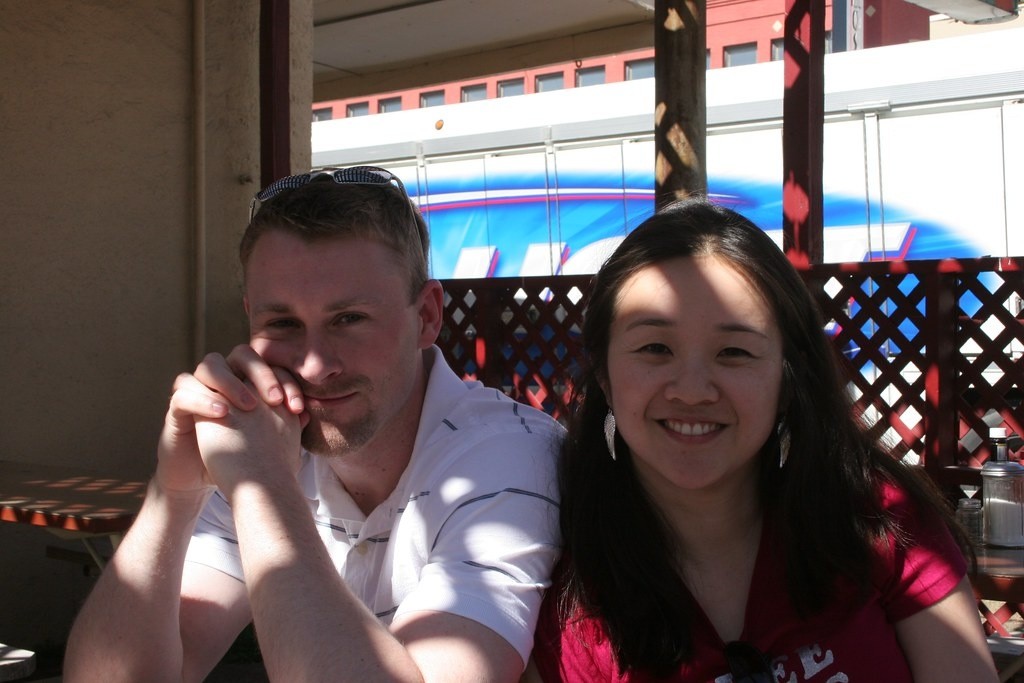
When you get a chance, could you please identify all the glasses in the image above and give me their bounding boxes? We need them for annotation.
[248,166,426,266]
[724,641,774,683]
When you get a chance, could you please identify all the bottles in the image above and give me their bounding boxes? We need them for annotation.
[989,427,1007,461]
[954,498,984,549]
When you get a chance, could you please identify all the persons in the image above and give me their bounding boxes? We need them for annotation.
[61,165,572,683]
[518,192,1005,683]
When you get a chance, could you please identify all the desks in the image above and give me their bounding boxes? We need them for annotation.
[959,540,1024,683]
[0,458,149,574]
